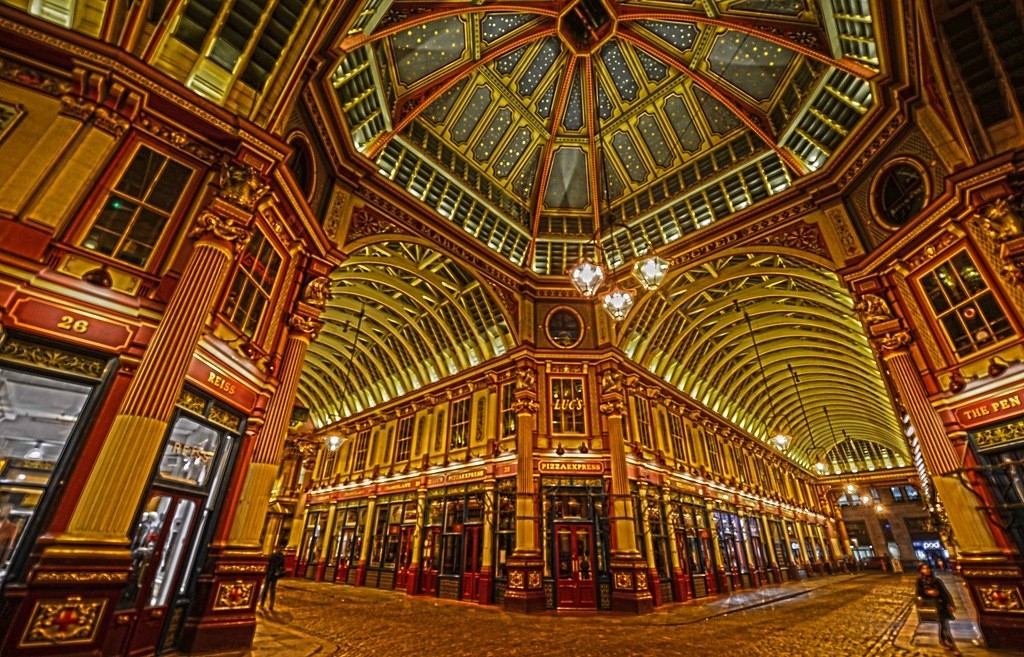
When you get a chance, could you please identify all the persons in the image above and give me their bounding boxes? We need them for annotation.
[934,555,949,572]
[883,553,893,576]
[843,554,864,574]
[915,563,964,655]
[257,544,286,611]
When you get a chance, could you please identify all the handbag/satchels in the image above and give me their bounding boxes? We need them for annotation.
[915,604,941,623]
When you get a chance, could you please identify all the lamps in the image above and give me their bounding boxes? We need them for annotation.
[322,307,365,452]
[788,364,830,475]
[569,24,670,323]
[823,406,858,493]
[744,313,794,451]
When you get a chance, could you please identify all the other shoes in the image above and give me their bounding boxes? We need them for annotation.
[951,647,961,657]
[939,641,949,650]
